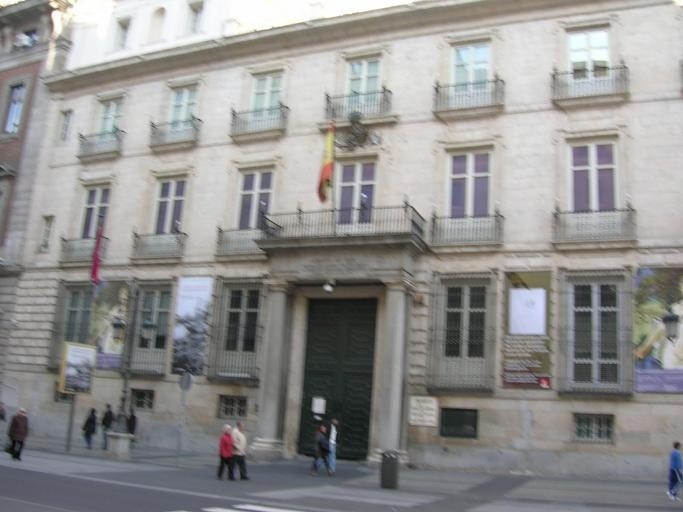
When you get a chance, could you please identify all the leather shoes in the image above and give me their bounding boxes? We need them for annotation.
[217,476,249,480]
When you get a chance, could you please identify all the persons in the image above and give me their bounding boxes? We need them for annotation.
[667,442,683,501]
[218,420,249,481]
[9,408,28,460]
[311,418,339,477]
[175,300,211,341]
[103,288,129,353]
[0,402,7,421]
[83,405,136,449]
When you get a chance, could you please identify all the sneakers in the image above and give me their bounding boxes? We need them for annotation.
[666,491,681,502]
[328,470,336,476]
[309,470,319,476]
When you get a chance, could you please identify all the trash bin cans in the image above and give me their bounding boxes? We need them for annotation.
[382,450,401,490]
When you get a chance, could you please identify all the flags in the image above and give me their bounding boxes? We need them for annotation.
[318,125,336,202]
[91,227,103,285]
